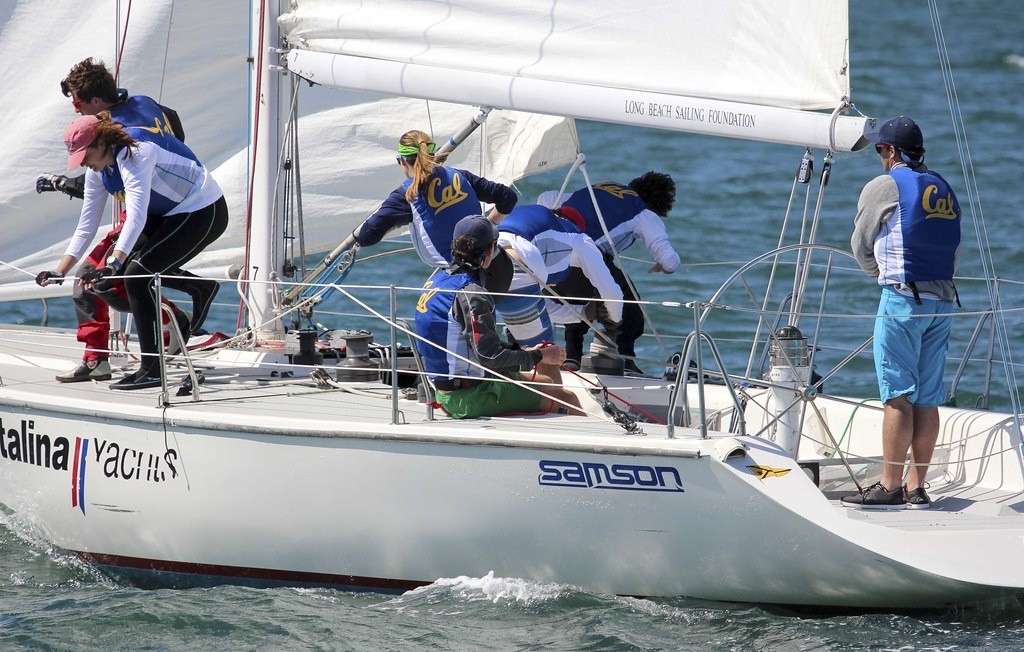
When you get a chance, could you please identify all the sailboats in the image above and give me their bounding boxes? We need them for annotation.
[2,0,1024,607]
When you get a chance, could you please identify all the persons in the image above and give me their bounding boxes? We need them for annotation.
[841,114,963,510]
[35,57,231,391]
[352,129,681,417]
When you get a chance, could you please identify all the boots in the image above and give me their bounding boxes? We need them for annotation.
[176,270,220,334]
[109,352,161,389]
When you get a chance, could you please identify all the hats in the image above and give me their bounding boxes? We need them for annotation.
[64,115,101,171]
[453,214,499,251]
[537,190,573,210]
[556,206,586,233]
[863,115,924,150]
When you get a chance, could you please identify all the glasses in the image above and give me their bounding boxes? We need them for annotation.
[396,155,408,165]
[72,99,85,109]
[875,143,890,154]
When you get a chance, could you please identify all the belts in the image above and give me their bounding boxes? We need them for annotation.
[434,378,481,390]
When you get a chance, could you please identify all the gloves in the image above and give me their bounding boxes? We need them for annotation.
[36,173,69,194]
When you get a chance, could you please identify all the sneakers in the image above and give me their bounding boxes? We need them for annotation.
[56,357,111,381]
[903,481,930,509]
[841,481,907,509]
[165,307,193,362]
[624,361,644,376]
[563,356,581,370]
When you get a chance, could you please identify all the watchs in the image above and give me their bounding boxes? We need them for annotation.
[106,256,122,270]
